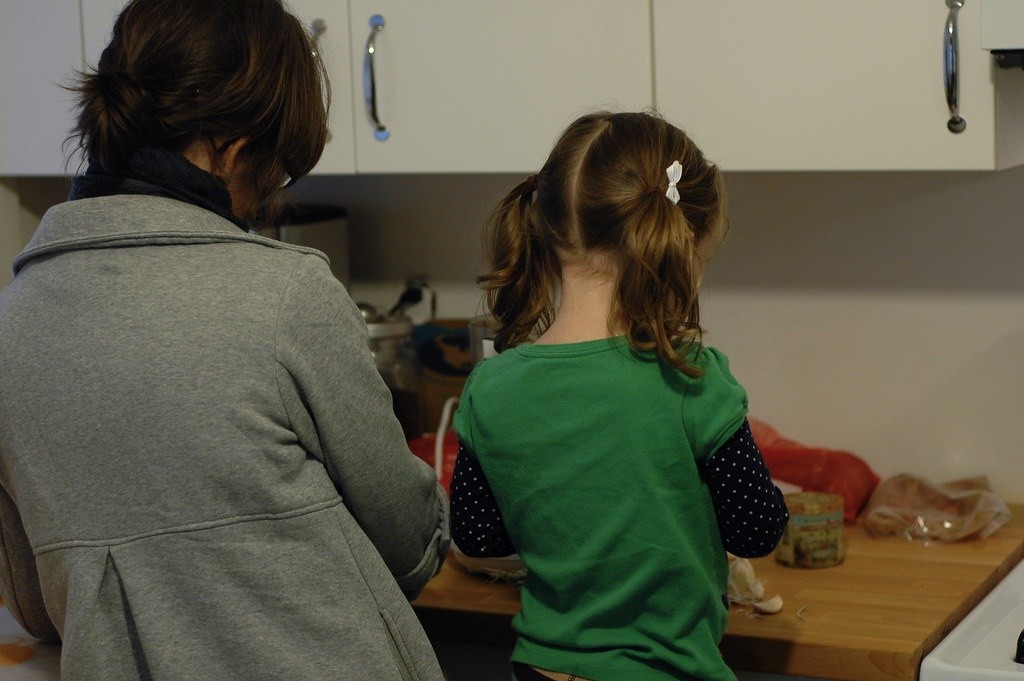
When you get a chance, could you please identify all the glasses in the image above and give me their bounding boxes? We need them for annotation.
[215,134,298,191]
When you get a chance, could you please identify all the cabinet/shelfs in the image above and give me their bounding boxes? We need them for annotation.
[1,0,1023,172]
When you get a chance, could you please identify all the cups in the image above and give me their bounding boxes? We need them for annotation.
[469,313,497,368]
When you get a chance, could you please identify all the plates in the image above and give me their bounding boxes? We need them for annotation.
[449,540,523,575]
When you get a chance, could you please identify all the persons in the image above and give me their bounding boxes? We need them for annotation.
[450,112,788,681]
[0,0,446,681]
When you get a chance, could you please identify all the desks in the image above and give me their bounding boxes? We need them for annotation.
[417,504,1024,681]
